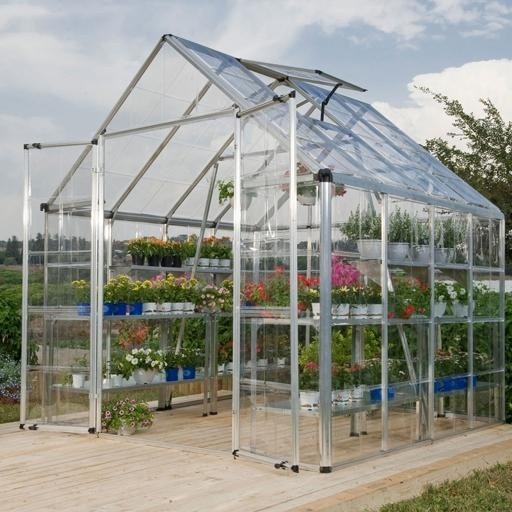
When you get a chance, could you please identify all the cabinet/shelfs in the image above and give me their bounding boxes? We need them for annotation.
[48,258,502,471]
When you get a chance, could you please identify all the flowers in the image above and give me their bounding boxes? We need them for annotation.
[126,236,235,257]
[215,178,235,203]
[98,397,157,434]
[300,349,492,389]
[240,264,484,319]
[67,271,306,313]
[72,340,285,377]
[281,162,346,199]
[336,203,466,249]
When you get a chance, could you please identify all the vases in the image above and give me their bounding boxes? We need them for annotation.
[229,194,253,211]
[131,251,231,267]
[354,238,456,266]
[75,303,198,315]
[295,189,318,206]
[70,356,286,387]
[296,376,480,406]
[117,423,138,439]
[310,302,475,321]
